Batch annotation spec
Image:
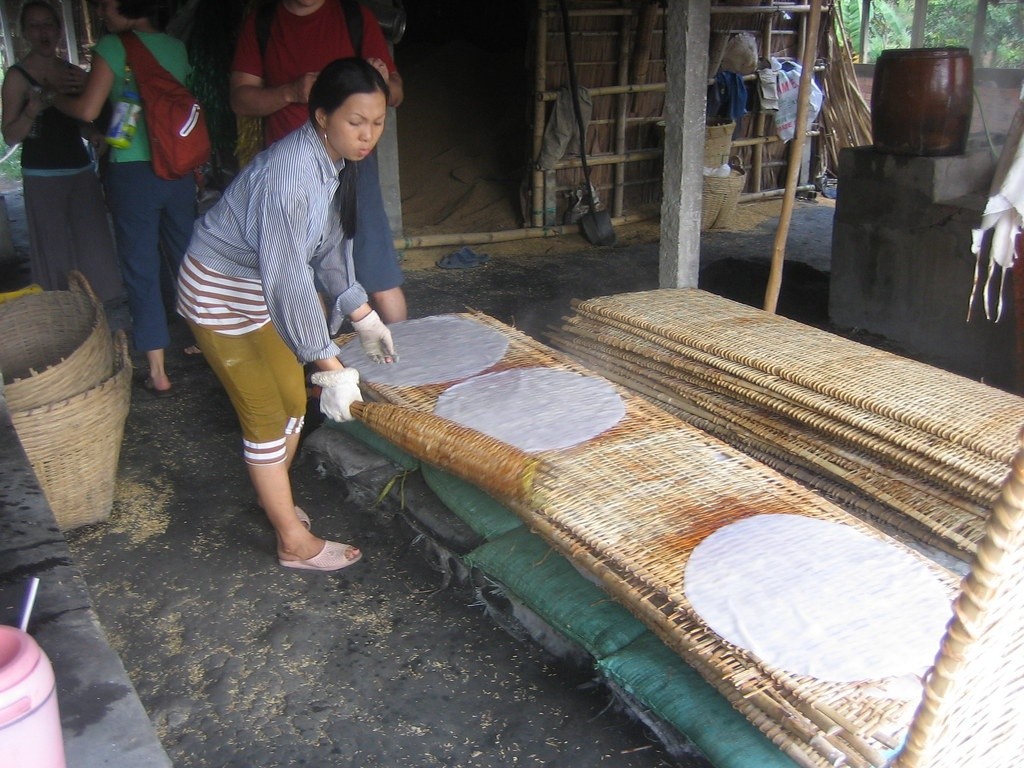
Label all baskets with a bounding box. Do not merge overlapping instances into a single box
[657,116,746,230]
[0,270,132,534]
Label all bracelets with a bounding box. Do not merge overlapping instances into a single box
[24,109,36,121]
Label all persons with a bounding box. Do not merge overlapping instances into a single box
[0,0,411,397]
[174,56,401,571]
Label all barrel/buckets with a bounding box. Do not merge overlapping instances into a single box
[870,46,976,157]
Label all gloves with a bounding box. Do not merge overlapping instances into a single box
[350,309,399,366]
[311,368,364,423]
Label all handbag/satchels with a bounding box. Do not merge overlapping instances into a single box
[118,30,211,181]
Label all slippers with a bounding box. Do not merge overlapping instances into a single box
[279,540,362,571]
[258,500,310,532]
[435,247,490,269]
[183,345,203,357]
[144,375,177,398]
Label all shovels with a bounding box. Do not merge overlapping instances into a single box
[559,2,615,246]
